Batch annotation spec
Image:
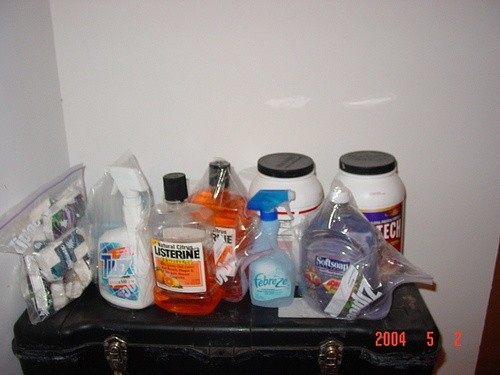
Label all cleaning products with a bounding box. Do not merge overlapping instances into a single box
[297,180,378,318]
[147,172,224,317]
[246,189,296,308]
[187,159,257,304]
[97,167,155,311]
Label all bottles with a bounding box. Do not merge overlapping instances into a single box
[333,150,406,279]
[247,152,324,286]
[149,172,221,316]
[298,189,378,319]
[189,160,249,302]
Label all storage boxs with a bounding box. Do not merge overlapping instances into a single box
[12,280,440,375]
